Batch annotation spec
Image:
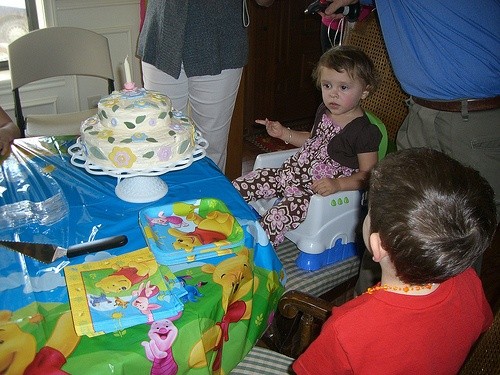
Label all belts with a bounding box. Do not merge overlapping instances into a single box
[411,95,500,112]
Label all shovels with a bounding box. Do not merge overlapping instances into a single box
[0,234,128,265]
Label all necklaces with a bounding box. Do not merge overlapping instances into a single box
[355,281,437,299]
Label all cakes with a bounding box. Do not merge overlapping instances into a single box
[79,87,195,169]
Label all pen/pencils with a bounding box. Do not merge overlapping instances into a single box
[255,120,267,126]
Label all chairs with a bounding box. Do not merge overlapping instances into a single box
[249,134,398,300]
[225,289,500,375]
[249,108,388,273]
[8,26,116,135]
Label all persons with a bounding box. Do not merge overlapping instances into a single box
[292,145,495,375]
[232,43,383,249]
[325,0,499,224]
[135,0,275,180]
[0,106,20,156]
[285,127,292,144]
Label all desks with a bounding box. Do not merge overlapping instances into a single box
[0,136,259,373]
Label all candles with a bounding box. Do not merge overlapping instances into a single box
[122,52,136,90]
